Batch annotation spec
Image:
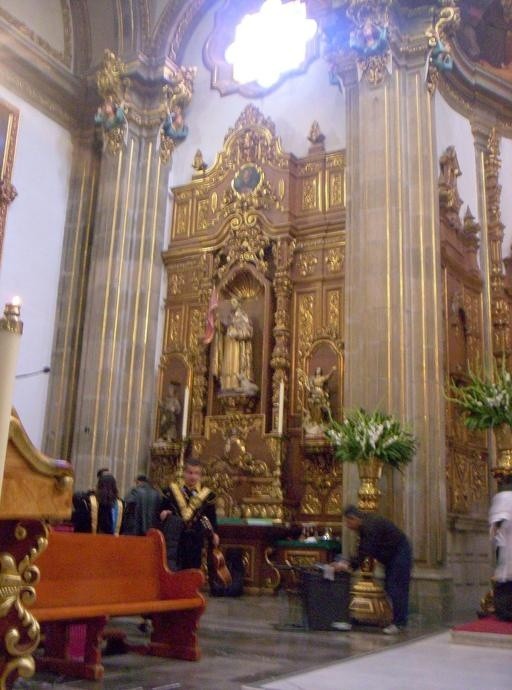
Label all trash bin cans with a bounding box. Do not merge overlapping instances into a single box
[300,566,351,631]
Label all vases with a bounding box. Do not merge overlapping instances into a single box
[357,457,385,512]
[491,423,512,478]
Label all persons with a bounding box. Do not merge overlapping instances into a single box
[217,295,256,389]
[71,468,111,531]
[326,506,412,633]
[160,456,221,572]
[488,476,512,620]
[79,474,126,536]
[125,471,161,536]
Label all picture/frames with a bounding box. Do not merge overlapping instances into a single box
[0,97,20,272]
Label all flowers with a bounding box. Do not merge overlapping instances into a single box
[441,350,512,432]
[319,394,421,475]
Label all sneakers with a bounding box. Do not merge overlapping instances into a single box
[383,624,400,634]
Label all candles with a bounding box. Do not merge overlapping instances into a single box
[182,385,189,440]
[278,379,284,434]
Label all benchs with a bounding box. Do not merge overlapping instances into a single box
[22,528,207,682]
[264,548,351,630]
